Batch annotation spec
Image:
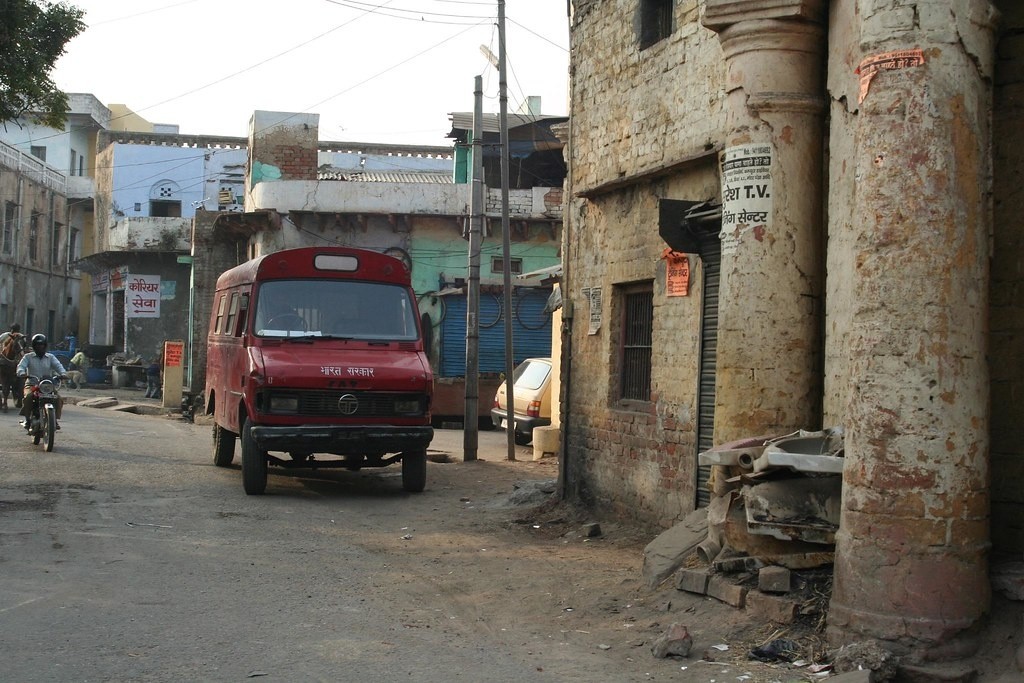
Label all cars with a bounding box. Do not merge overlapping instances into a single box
[490,356,553,448]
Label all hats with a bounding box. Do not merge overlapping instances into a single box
[8,323,21,330]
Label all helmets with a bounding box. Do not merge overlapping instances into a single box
[32,334,47,357]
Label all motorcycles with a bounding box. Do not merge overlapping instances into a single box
[19,373,68,452]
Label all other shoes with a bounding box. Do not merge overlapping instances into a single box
[23,420,29,428]
[56,421,60,429]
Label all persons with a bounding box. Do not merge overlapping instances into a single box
[15,334,68,430]
[69,349,87,389]
[144,364,161,398]
[261,292,304,331]
[0,323,26,354]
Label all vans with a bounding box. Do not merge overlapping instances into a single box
[204,245,435,497]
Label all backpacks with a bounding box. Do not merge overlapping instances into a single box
[7,333,27,360]
